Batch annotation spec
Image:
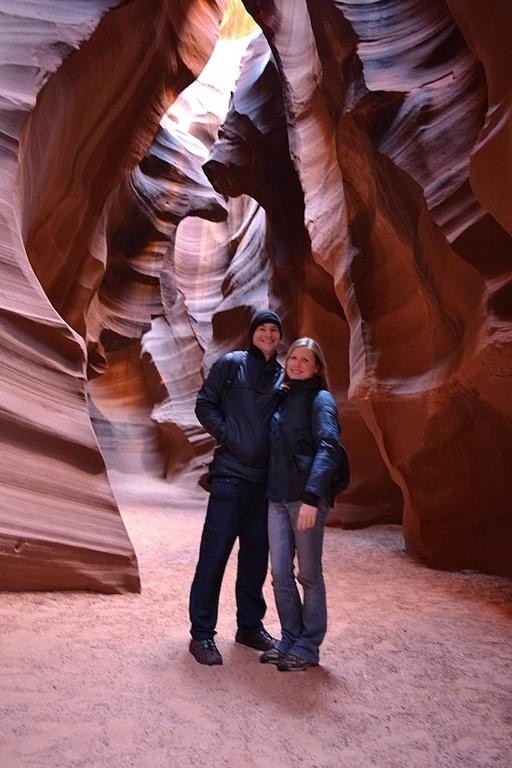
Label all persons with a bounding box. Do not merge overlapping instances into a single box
[260,337,349,671]
[190,308,280,664]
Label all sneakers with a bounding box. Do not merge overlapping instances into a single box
[190,638,222,665]
[260,649,319,671]
[236,631,277,649]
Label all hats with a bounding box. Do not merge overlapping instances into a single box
[250,310,283,340]
